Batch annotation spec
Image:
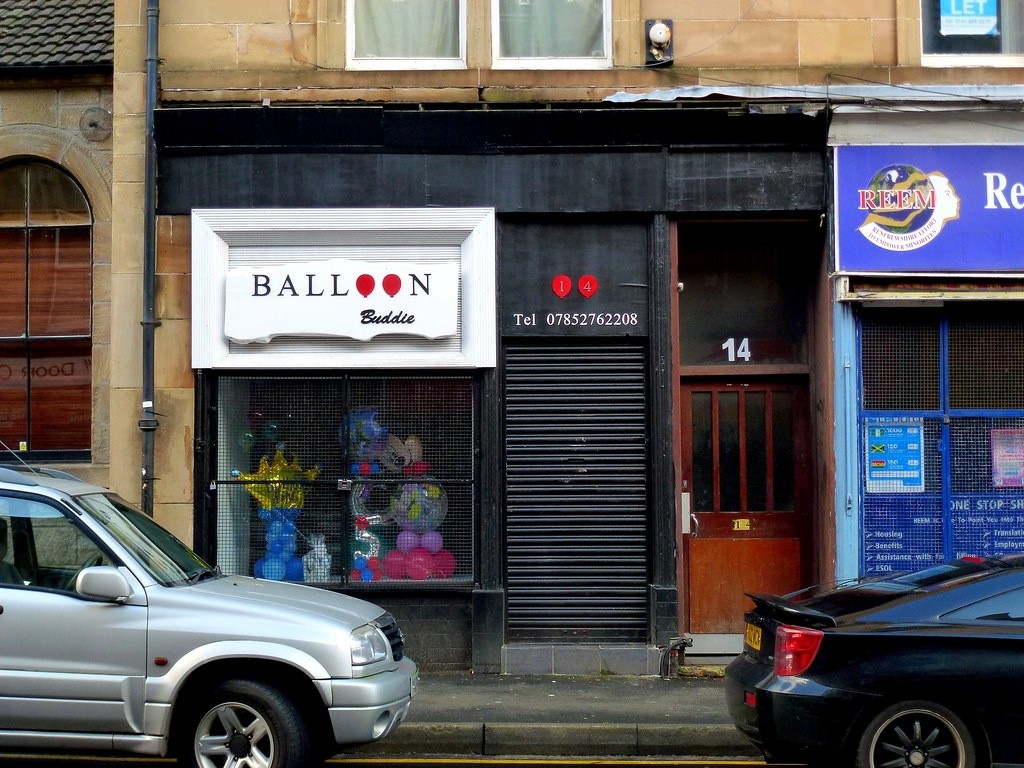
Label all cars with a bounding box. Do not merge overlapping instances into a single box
[724,553,1024,768]
[1,464,419,768]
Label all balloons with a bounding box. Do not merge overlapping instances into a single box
[232,445,321,581]
[338,406,455,580]
